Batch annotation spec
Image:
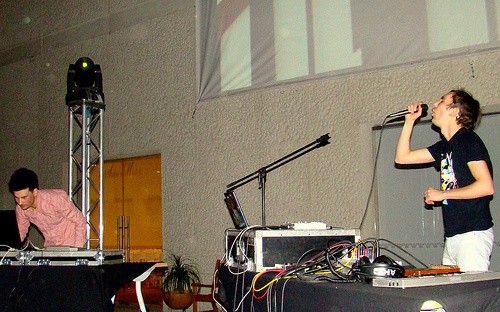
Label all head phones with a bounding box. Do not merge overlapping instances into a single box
[352,255,405,283]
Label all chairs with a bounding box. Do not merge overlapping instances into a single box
[190,259,221,312]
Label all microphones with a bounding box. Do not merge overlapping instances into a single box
[386,104,428,118]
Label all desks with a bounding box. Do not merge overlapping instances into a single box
[219,264,500,312]
[1,266,167,312]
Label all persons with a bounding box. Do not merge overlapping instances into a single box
[8,168,87,249]
[394,87,495,271]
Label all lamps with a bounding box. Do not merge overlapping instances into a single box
[67,56,104,95]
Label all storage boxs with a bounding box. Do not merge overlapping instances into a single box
[227,228,362,272]
[0,249,127,266]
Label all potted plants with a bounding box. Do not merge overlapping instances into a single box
[164,251,195,309]
